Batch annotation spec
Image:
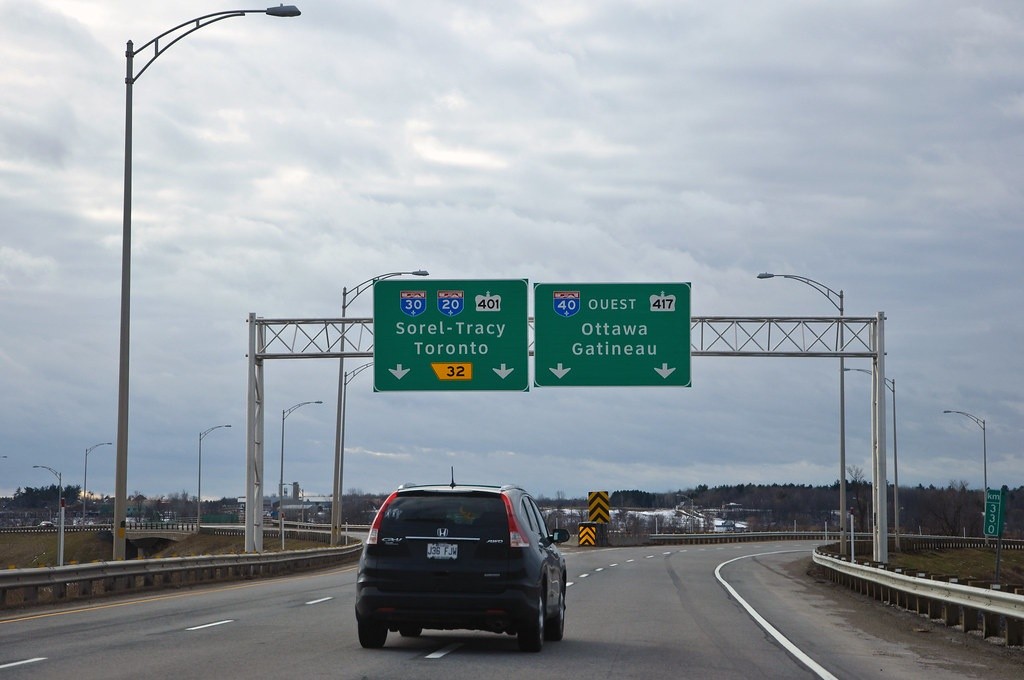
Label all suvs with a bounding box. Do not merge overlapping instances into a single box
[353,483,571,654]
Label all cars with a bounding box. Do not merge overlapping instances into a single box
[37,521,54,527]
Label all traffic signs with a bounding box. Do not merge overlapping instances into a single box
[370,278,533,393]
[529,280,693,388]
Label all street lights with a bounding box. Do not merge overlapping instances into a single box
[675,494,694,533]
[842,367,902,552]
[33,464,61,565]
[82,442,113,525]
[755,272,848,556]
[279,401,324,548]
[329,269,435,545]
[942,410,986,513]
[195,425,232,532]
[114,5,301,562]
